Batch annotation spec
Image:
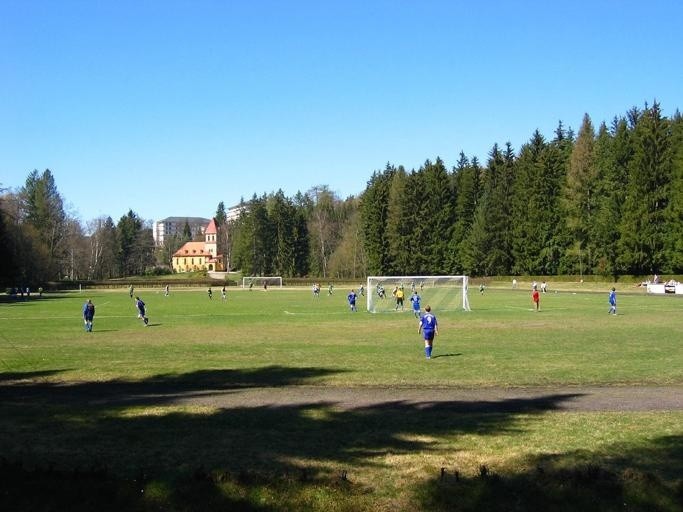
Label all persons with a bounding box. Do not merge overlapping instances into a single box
[417,305,438,359]
[532,279,537,292]
[312,283,320,299]
[134,297,148,325]
[540,281,546,293]
[409,291,421,318]
[37,287,43,296]
[511,278,516,289]
[347,289,356,311]
[129,284,133,297]
[375,279,425,301]
[220,285,227,300]
[327,282,334,296]
[607,287,618,314]
[207,287,212,300]
[394,288,404,311]
[82,299,93,332]
[358,282,364,297]
[164,284,168,296]
[532,288,540,312]
[479,282,484,294]
[249,282,254,291]
[262,282,266,292]
